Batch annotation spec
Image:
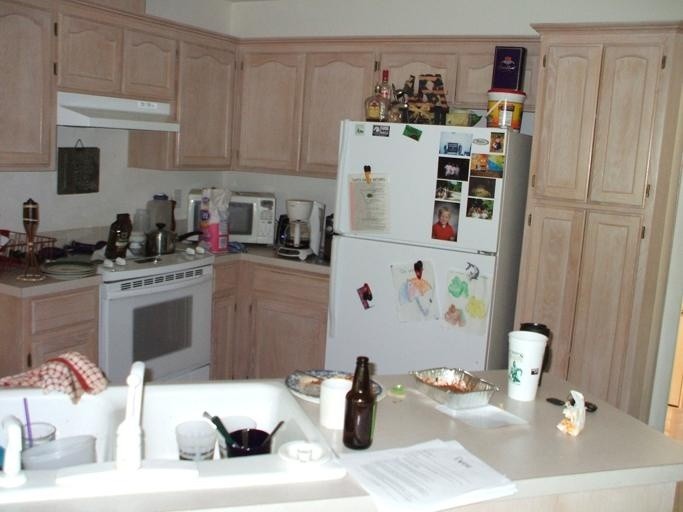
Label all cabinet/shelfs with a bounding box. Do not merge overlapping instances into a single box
[125,22,239,172]
[236,35,379,181]
[510,20,681,427]
[55,0,181,123]
[374,34,541,114]
[211,259,329,382]
[0,1,57,173]
[0,285,102,380]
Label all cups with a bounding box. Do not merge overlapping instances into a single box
[175,419,218,464]
[506,329,549,403]
[22,420,58,452]
[317,375,348,434]
[217,421,273,458]
[517,317,549,393]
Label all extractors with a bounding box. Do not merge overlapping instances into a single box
[54,90,180,132]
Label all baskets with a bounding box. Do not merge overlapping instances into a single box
[1,229,58,272]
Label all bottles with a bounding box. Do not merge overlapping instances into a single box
[343,357,378,452]
[144,191,174,230]
[134,208,150,230]
[363,69,392,121]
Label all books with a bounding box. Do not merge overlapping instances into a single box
[345,437,517,511]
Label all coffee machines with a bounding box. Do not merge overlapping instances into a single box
[276,198,324,258]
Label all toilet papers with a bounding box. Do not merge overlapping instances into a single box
[319,378,353,432]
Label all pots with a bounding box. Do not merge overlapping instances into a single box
[144,224,203,255]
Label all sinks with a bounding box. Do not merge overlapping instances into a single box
[1,379,348,508]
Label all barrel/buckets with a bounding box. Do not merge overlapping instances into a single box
[146,193,174,232]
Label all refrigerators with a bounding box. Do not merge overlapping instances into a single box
[322,119,532,380]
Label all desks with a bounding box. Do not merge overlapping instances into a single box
[279,367,681,512]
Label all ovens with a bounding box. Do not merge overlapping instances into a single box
[100,267,213,380]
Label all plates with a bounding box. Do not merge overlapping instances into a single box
[40,261,96,281]
[284,368,387,407]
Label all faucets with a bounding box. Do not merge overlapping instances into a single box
[116,360,146,472]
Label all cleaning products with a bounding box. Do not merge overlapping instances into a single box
[197,186,232,252]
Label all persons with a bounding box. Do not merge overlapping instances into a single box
[431,136,503,243]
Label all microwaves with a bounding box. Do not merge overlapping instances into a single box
[186,184,276,249]
